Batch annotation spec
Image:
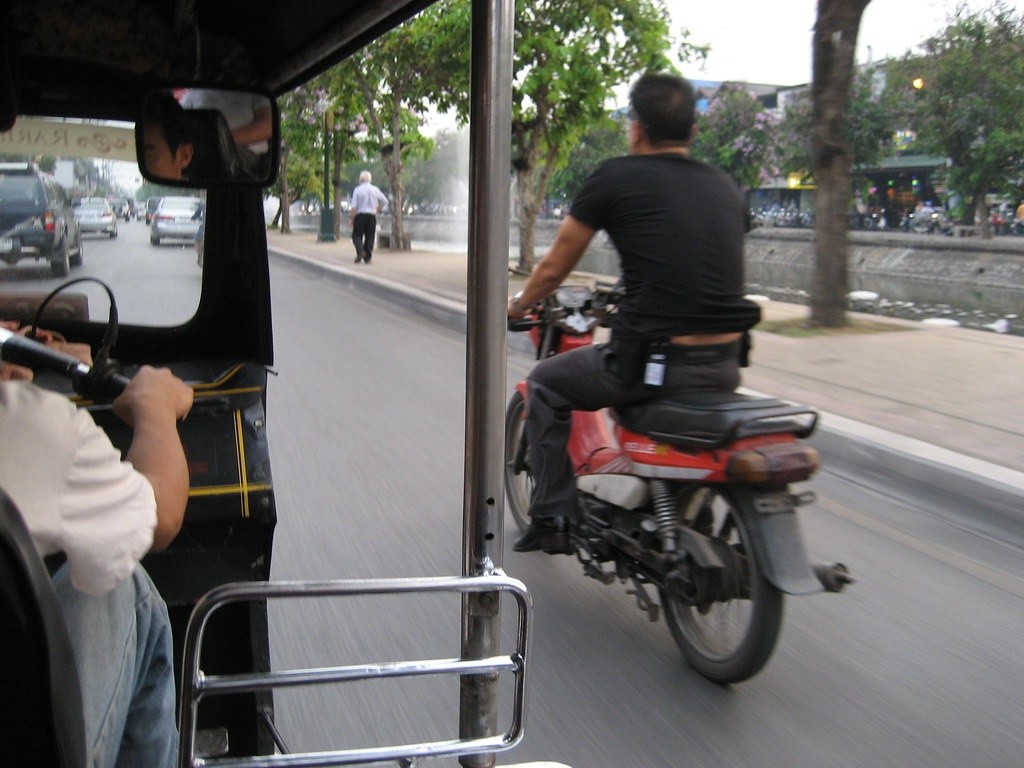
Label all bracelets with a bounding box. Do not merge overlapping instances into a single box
[510,289,535,312]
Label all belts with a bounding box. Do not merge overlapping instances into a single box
[611,339,742,364]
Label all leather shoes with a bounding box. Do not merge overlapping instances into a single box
[512,515,570,551]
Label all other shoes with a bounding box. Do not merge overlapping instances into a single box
[355,250,370,262]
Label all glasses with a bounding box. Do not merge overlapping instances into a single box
[142,146,177,167]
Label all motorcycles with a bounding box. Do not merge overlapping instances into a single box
[506,280,856,685]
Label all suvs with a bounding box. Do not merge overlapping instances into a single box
[0,160,84,278]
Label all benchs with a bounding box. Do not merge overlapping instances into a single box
[953,225,996,239]
[376,230,411,251]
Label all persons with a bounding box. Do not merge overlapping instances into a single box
[348,170,388,265]
[507,73,761,553]
[191,189,206,265]
[0,364,193,767]
[756,186,1024,233]
[141,89,272,181]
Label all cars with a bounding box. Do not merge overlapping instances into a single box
[109,196,146,223]
[145,198,161,224]
[149,195,202,247]
[70,197,118,239]
